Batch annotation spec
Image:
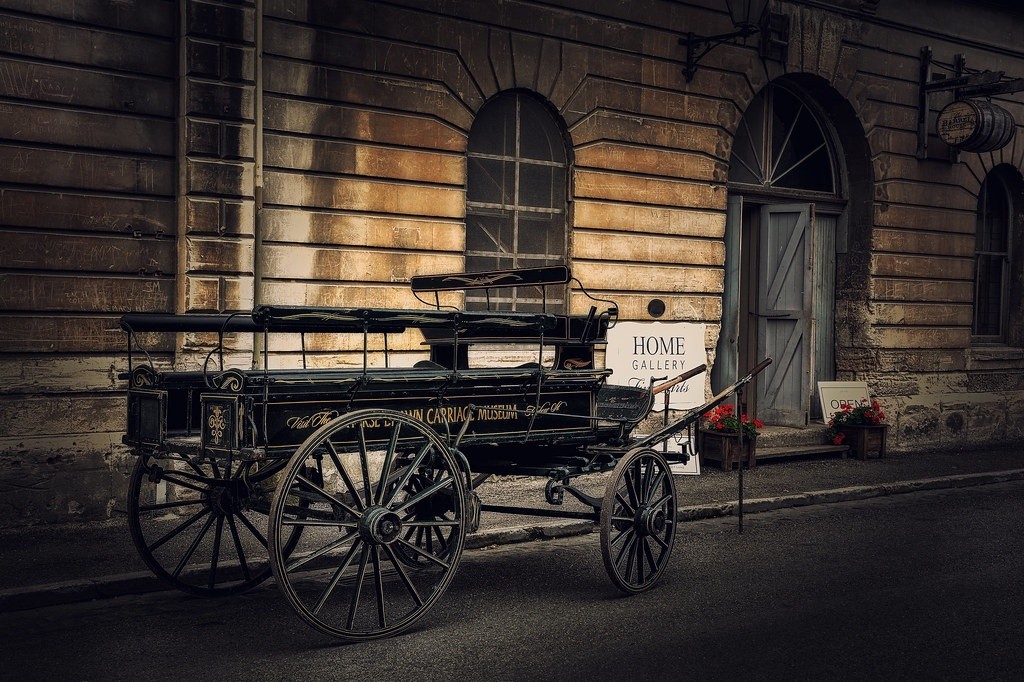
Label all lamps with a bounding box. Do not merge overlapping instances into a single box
[678,0,770,84]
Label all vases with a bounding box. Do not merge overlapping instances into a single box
[696,429,761,472]
[837,424,890,461]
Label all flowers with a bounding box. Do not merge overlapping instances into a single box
[703,405,763,434]
[825,399,885,446]
